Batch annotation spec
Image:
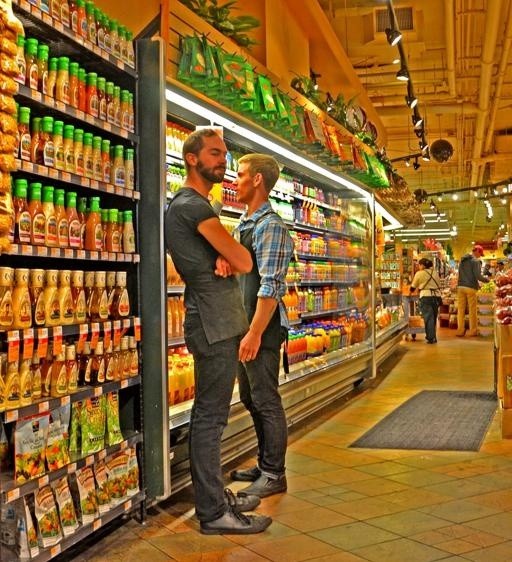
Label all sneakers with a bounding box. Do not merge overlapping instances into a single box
[230,464,261,481]
[426,336,436,344]
[223,488,261,511]
[237,474,287,498]
[200,508,272,535]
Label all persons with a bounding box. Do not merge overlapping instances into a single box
[497,262,504,277]
[225,154,295,499]
[165,129,272,535]
[484,264,492,276]
[410,258,442,344]
[456,246,490,338]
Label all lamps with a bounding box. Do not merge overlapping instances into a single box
[418,140,427,150]
[429,193,443,222]
[412,156,421,170]
[405,95,419,109]
[396,64,409,81]
[385,27,403,46]
[411,114,423,127]
[422,153,430,162]
[414,129,425,138]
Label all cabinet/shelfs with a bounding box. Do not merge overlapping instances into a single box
[166,112,371,498]
[375,195,455,374]
[0,0,150,562]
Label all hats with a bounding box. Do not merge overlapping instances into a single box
[473,245,484,256]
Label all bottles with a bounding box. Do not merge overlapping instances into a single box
[10,92,134,192]
[0,266,129,319]
[8,0,136,69]
[375,302,405,334]
[1,170,136,255]
[11,7,133,131]
[0,335,140,411]
[167,120,368,407]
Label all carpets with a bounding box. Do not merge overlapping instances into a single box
[347,390,497,452]
